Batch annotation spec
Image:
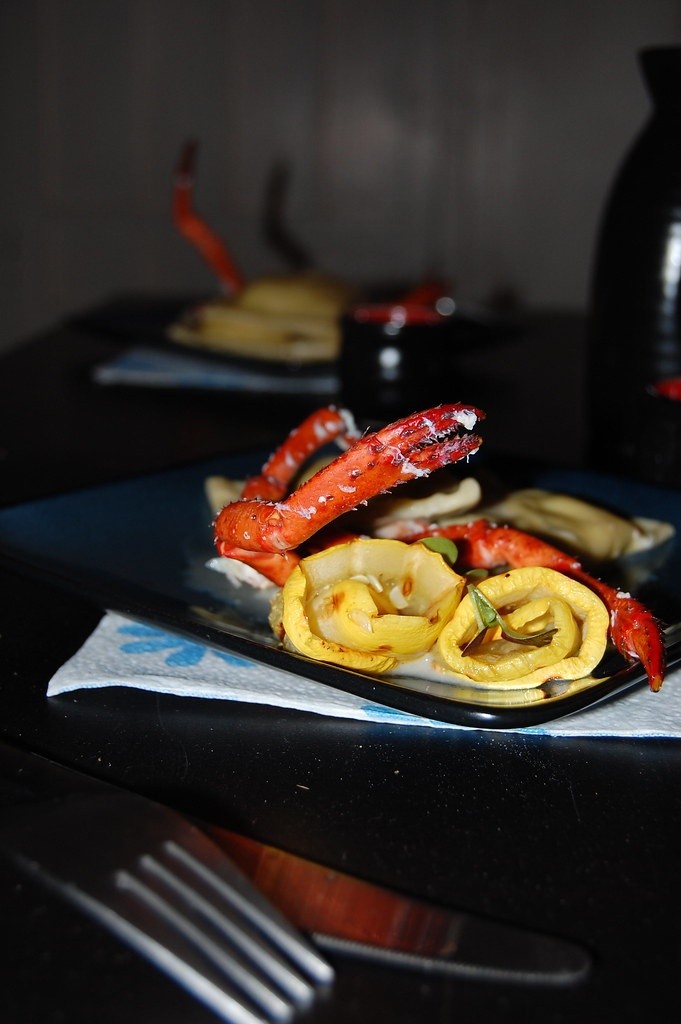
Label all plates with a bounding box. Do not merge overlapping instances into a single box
[66,294,336,374]
[0,451,681,726]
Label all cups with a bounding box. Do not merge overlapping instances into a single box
[333,281,516,498]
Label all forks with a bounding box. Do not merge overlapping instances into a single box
[0,746,334,1023]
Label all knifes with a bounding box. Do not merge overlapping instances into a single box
[192,820,591,978]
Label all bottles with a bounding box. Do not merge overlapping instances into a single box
[586,38,681,475]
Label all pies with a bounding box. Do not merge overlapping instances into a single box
[194,277,350,346]
[282,539,466,674]
[441,560,608,687]
